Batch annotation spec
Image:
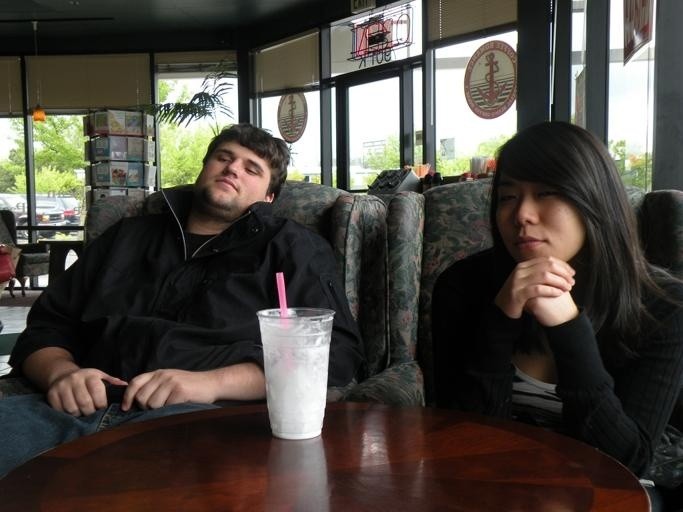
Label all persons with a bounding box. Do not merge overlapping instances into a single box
[427,121,681,511]
[0,123,367,480]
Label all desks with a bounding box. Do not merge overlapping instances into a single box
[83,410,647,495]
[0,398,652,512]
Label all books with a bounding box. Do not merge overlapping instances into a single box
[80,108,159,210]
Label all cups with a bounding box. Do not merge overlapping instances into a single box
[255,306,337,441]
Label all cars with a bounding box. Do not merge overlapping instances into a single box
[0,192,82,241]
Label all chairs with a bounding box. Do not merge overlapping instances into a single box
[385,180,683,400]
[0,217,50,295]
[83,194,381,400]
